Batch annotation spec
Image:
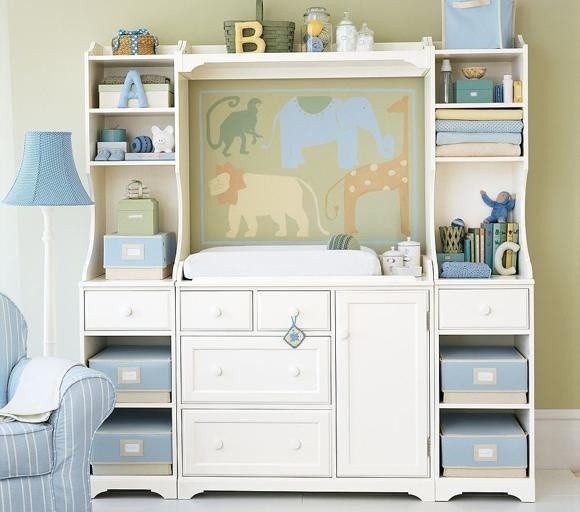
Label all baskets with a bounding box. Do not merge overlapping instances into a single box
[223,0,296,54]
[112,32,159,55]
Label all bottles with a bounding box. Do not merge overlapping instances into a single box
[439,58,454,103]
[381,235,423,279]
[302,7,332,52]
[335,12,376,52]
[502,73,513,103]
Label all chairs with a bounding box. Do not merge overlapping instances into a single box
[0,291,116,512]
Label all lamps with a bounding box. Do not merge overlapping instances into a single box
[1,130,95,358]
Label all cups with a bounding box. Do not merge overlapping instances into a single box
[101,129,126,141]
[438,225,464,253]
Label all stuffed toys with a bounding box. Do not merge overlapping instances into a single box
[480,190,516,223]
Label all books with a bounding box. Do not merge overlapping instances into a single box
[466,223,519,275]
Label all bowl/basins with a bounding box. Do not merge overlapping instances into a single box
[461,67,488,79]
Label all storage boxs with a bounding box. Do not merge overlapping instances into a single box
[441,0,517,50]
